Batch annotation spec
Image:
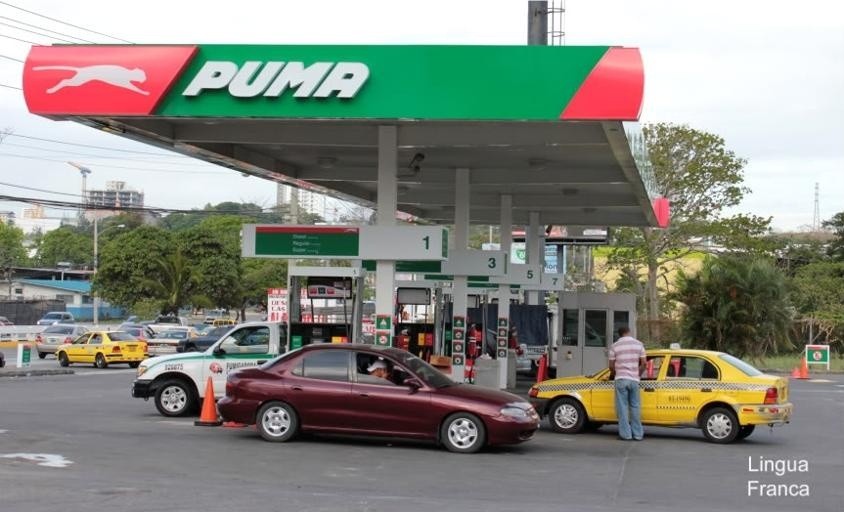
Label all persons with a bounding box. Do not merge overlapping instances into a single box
[607,327,649,442]
[383,359,405,384]
[367,361,389,381]
[467,315,488,347]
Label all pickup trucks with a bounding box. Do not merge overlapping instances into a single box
[131,319,297,417]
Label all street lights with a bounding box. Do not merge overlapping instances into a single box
[92,224,126,326]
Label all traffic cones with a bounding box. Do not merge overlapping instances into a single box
[192,376,223,427]
[796,357,811,379]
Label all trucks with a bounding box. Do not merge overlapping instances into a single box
[467,305,603,376]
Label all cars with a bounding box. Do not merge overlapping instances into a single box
[483,328,533,373]
[527,342,794,446]
[0,315,14,327]
[216,342,542,453]
[34,310,239,370]
